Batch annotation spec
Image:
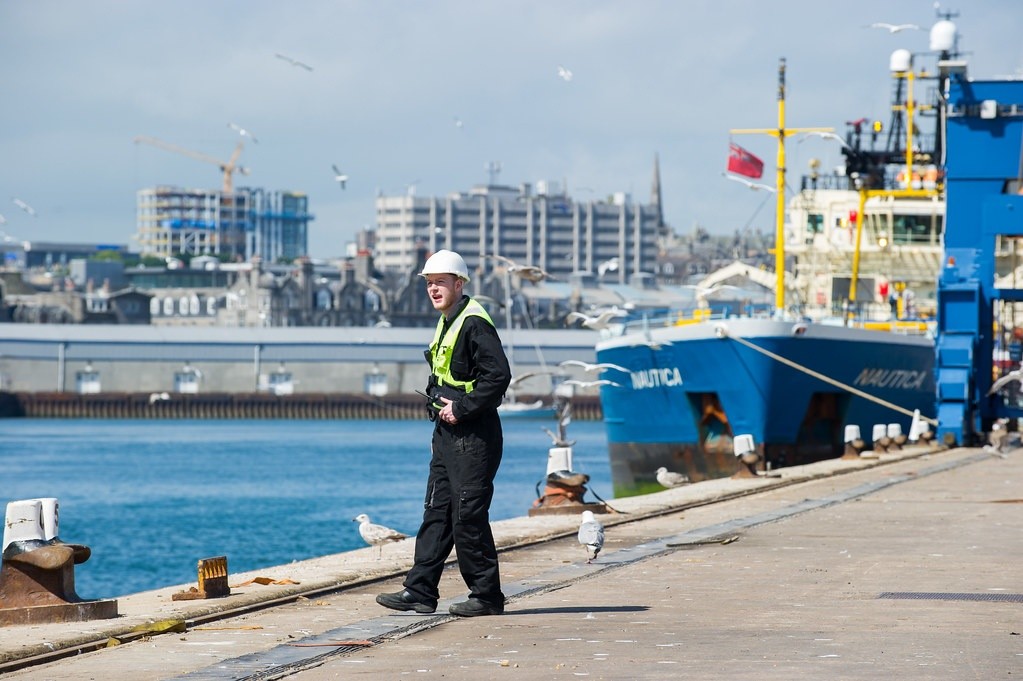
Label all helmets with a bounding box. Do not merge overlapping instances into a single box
[416,249,470,286]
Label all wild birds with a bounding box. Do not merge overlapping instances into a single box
[332,164,349,190]
[864,22,931,36]
[556,358,634,375]
[557,66,572,81]
[226,121,258,143]
[148,392,170,405]
[352,513,412,561]
[559,378,624,391]
[562,310,620,330]
[654,467,691,489]
[478,253,559,286]
[13,199,37,218]
[722,173,777,195]
[797,132,855,153]
[507,371,536,391]
[679,283,740,300]
[276,53,313,72]
[597,257,621,276]
[632,339,675,352]
[577,510,606,564]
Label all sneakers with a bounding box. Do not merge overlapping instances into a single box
[375,589,437,613]
[449,597,504,615]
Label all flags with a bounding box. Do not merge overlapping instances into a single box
[727,142,764,179]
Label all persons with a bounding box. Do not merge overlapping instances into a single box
[376,249,511,618]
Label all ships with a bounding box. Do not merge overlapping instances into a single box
[595,0,1022,500]
